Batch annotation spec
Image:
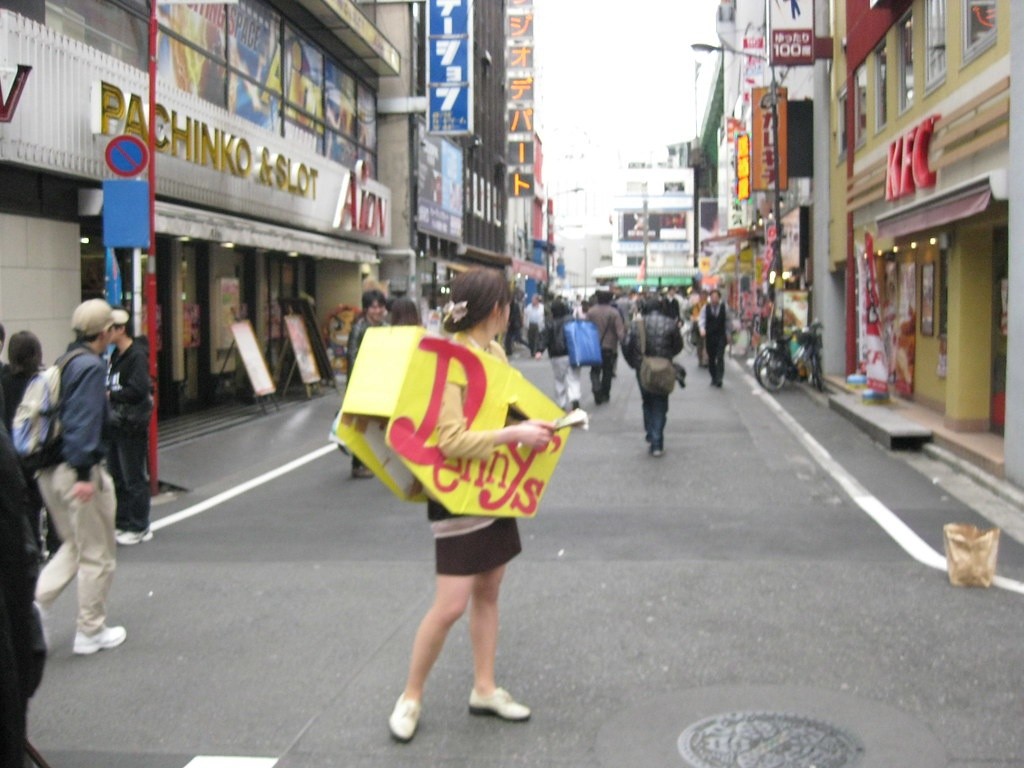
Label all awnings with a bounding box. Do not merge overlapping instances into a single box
[77,187,382,266]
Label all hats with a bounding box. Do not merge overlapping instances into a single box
[71,299,129,336]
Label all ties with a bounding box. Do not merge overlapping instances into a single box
[712,306,717,315]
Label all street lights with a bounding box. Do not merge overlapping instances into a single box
[690,43,784,338]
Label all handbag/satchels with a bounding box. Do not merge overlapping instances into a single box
[640,357,676,395]
[565,308,603,368]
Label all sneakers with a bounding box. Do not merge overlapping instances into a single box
[74,626,127,655]
[469,687,532,721]
[117,526,154,545]
[390,694,420,741]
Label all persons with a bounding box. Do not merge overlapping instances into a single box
[690,291,729,387]
[390,270,556,742]
[502,289,545,361]
[549,286,619,411]
[619,285,687,456]
[111,308,152,545]
[0,299,125,768]
[346,289,422,478]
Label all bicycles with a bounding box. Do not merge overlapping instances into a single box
[752,317,824,392]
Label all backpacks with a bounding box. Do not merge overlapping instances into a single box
[12,349,88,458]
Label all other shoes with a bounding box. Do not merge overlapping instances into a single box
[595,395,610,405]
[352,467,374,479]
[712,380,722,387]
[652,444,662,456]
[646,434,652,441]
[572,400,579,409]
